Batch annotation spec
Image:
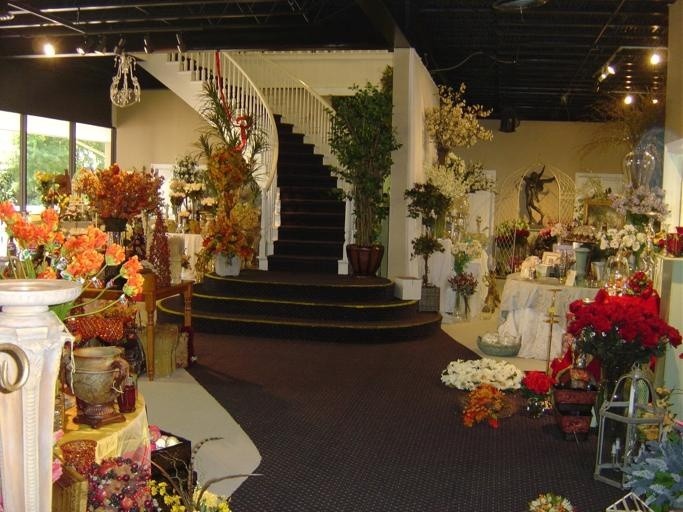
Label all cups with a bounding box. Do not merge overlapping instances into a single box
[529,269,538,281]
[559,264,566,282]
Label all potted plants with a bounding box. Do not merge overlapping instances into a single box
[323,64,404,280]
[404,182,454,312]
[193,80,273,182]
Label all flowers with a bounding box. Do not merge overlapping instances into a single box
[494,218,530,279]
[450,240,483,275]
[560,415,591,442]
[623,435,683,511]
[564,287,683,381]
[440,357,525,393]
[534,223,563,259]
[557,387,597,415]
[88,457,154,512]
[150,437,266,512]
[0,201,145,323]
[522,370,558,397]
[599,226,647,262]
[423,82,499,239]
[167,145,261,266]
[460,383,517,427]
[529,495,574,512]
[638,385,683,442]
[448,272,477,299]
[613,185,672,223]
[32,165,166,225]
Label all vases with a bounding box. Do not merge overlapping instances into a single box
[463,422,500,468]
[525,396,554,420]
[101,218,128,250]
[454,295,468,323]
[215,250,241,278]
[598,365,625,449]
